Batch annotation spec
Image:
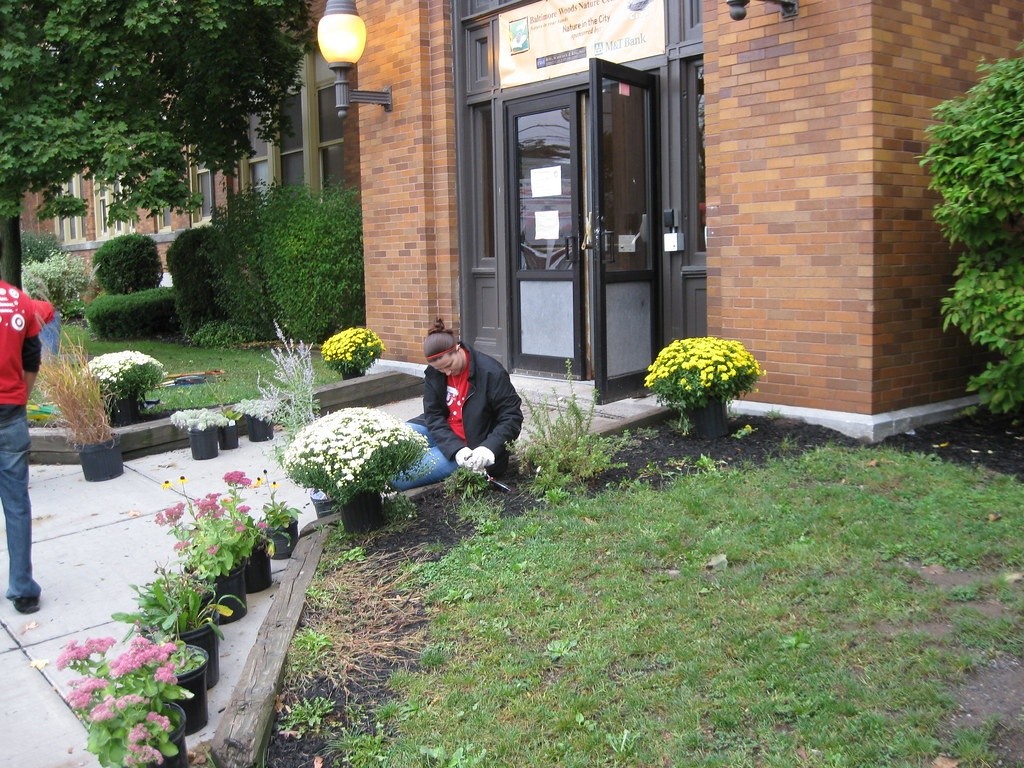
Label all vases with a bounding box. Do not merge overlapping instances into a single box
[248,419,274,442]
[108,388,139,424]
[335,491,385,534]
[342,373,366,380]
[180,556,248,625]
[163,644,210,736]
[186,428,220,461]
[261,519,299,560]
[245,541,272,595]
[74,434,123,482]
[139,609,220,691]
[120,701,189,768]
[310,488,340,520]
[693,395,729,439]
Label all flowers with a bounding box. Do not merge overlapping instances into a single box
[76,350,168,413]
[231,398,280,422]
[170,407,230,432]
[255,319,440,515]
[54,469,301,768]
[644,336,767,411]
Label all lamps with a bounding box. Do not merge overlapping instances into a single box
[318,0,392,120]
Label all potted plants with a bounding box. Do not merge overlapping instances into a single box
[216,407,243,449]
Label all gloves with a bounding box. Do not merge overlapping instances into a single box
[455,446,495,474]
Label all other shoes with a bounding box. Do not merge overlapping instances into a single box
[13,594,40,614]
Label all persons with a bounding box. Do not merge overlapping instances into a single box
[369,317,523,490]
[0,240,43,614]
[30,299,60,364]
